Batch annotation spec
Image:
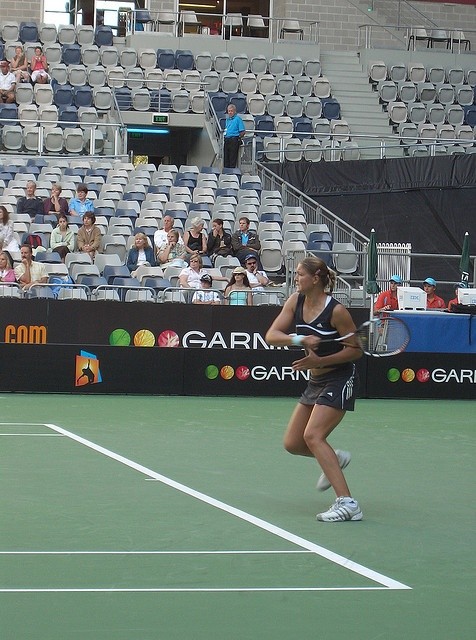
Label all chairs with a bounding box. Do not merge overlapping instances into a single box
[446,28,471,53]
[131,8,155,31]
[426,28,452,50]
[155,9,175,33]
[247,14,269,37]
[222,13,244,38]
[1,41,332,94]
[367,59,476,156]
[4,23,114,44]
[406,26,432,52]
[209,95,361,160]
[176,10,202,35]
[0,160,361,303]
[280,18,303,40]
[0,87,209,157]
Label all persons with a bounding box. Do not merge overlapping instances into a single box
[12,46,30,83]
[178,254,230,303]
[245,254,269,288]
[0,61,16,103]
[231,217,263,271]
[224,266,252,306]
[0,206,13,249]
[192,274,220,305]
[14,244,49,290]
[0,252,15,282]
[373,275,401,324]
[51,214,74,261]
[77,211,101,260]
[44,185,68,215]
[68,186,94,217]
[31,46,51,84]
[224,104,245,168]
[127,233,158,277]
[448,282,465,312]
[154,216,184,252]
[422,278,446,308]
[184,217,207,263]
[156,229,187,272]
[207,218,231,263]
[17,181,42,223]
[266,257,364,522]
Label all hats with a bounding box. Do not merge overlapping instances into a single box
[199,274,211,286]
[0,62,9,67]
[422,278,436,287]
[232,267,246,275]
[244,255,257,260]
[453,282,464,288]
[389,275,401,284]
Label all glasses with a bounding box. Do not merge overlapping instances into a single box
[189,260,196,262]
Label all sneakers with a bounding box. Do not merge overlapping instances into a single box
[315,496,363,521]
[316,449,349,492]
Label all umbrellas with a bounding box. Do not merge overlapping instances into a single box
[459,232,472,288]
[366,228,380,351]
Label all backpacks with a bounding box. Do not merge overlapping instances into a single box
[26,235,42,249]
